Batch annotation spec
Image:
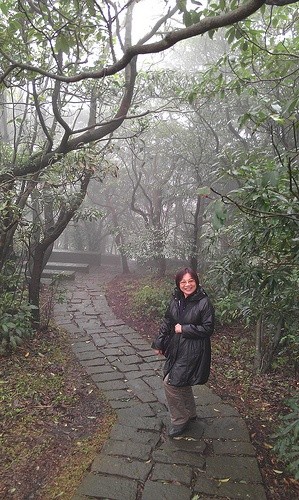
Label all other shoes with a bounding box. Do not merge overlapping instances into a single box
[169,422,186,437]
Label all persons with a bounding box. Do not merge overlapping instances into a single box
[151,267,215,438]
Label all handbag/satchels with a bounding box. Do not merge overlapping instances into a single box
[161,327,176,358]
